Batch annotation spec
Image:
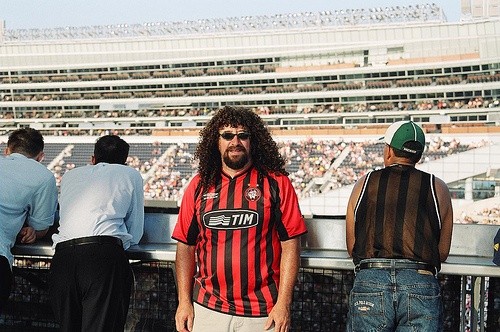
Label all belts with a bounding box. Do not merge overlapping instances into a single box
[55,236,121,250]
[360,262,436,271]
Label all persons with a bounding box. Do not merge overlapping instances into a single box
[346,120,453,332]
[0,93,494,221]
[48,135,144,332]
[172,106,308,332]
[0,128,58,315]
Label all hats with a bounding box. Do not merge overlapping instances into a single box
[375,120,425,154]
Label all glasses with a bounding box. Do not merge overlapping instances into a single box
[220,132,250,140]
